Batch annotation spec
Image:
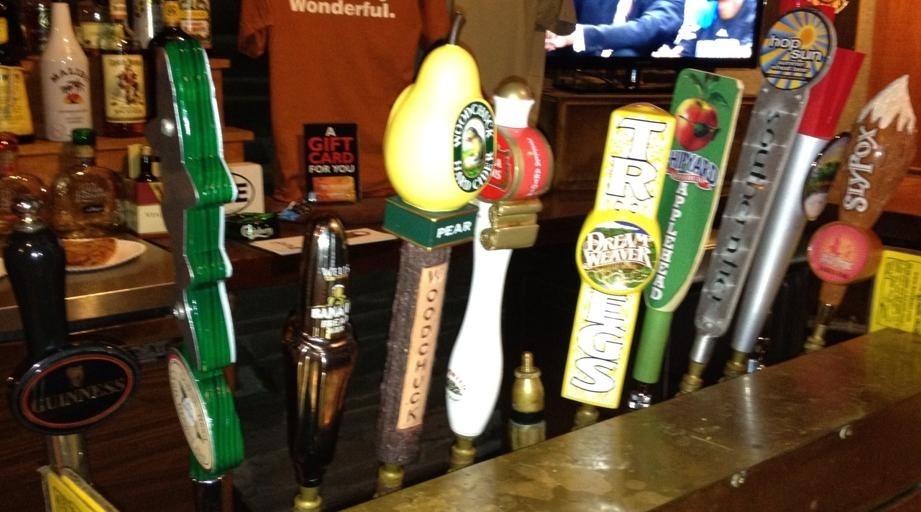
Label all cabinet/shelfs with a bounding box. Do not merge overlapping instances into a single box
[0,52,256,233]
[534,83,758,223]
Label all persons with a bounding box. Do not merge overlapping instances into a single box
[545,0,685,57]
[682,0,756,58]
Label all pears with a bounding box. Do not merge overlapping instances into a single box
[382,13,498,213]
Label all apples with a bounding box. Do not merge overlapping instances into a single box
[674,97,718,151]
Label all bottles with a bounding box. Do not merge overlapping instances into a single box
[98,0,148,136]
[41,3,95,144]
[160,1,215,58]
[135,145,160,183]
[0,0,36,145]
[54,126,127,239]
[0,129,56,256]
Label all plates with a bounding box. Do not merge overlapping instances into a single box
[62,237,148,274]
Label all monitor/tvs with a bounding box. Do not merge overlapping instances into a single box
[545,0,763,94]
